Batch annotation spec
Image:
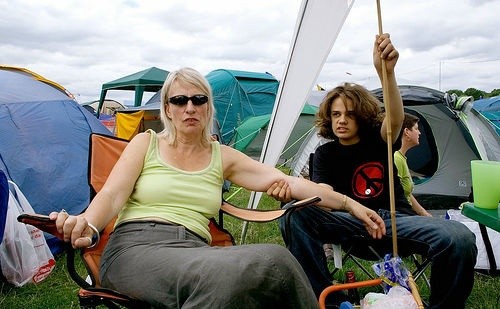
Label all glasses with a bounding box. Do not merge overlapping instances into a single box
[169,94,208,106]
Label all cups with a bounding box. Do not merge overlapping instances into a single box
[471,159,500,210]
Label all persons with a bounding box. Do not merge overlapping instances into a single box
[267,34,478,309]
[49,67,386,309]
[393,113,435,218]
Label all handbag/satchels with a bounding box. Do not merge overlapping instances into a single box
[0,180,56,287]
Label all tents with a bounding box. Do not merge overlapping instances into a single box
[286,85,500,218]
[203,69,280,146]
[114,87,232,193]
[0,68,114,275]
[77,99,127,122]
[96,67,171,119]
[226,103,320,167]
[470,94,500,136]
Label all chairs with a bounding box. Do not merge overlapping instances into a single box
[16,132,431,309]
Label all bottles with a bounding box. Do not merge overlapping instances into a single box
[345,271,360,305]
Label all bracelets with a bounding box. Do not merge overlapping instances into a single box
[342,193,349,212]
[83,222,101,249]
